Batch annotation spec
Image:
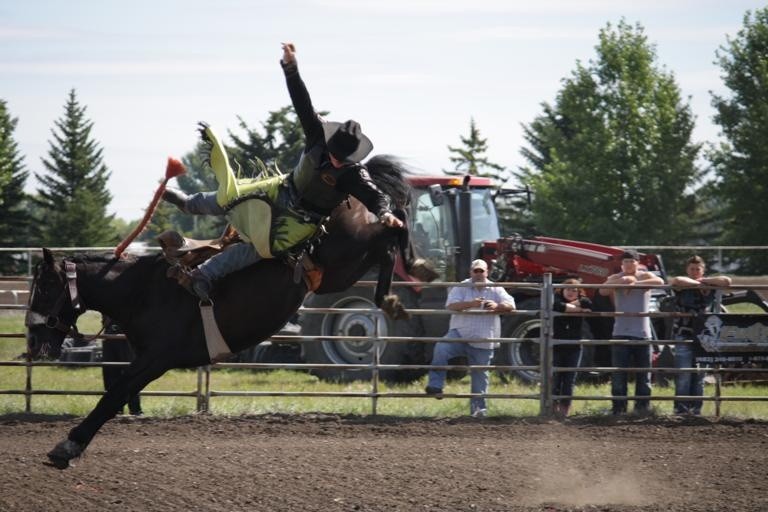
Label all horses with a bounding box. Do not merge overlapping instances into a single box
[23,152,443,471]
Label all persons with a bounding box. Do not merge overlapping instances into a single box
[162,42,404,303]
[553,279,593,417]
[598,250,664,415]
[426,259,516,418]
[671,255,731,414]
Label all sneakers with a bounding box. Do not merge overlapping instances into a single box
[161,187,187,214]
[552,403,571,417]
[191,267,209,302]
[424,385,443,399]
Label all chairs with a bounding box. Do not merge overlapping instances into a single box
[415,223,448,267]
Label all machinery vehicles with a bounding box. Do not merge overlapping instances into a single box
[228,165,768,395]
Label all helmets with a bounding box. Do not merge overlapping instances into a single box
[470,258,489,273]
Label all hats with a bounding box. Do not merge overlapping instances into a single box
[621,250,640,261]
[320,120,374,165]
